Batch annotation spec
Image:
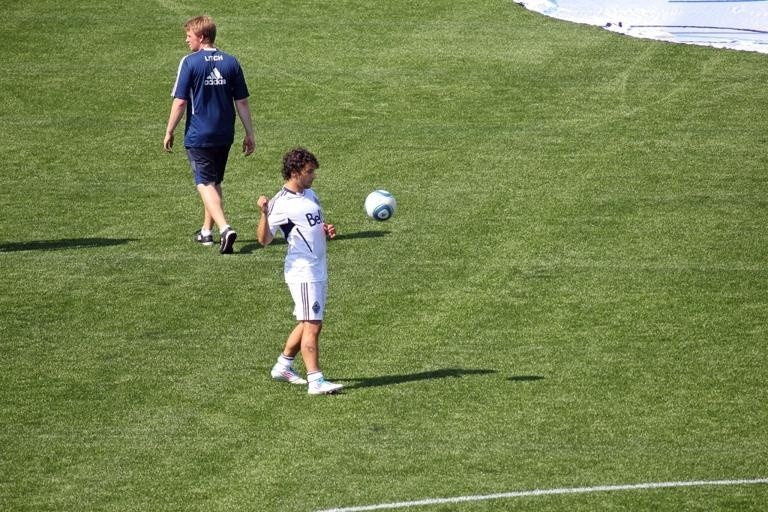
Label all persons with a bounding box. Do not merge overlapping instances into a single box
[258,147,345,395]
[162,15,256,255]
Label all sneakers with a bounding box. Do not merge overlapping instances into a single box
[308,382,343,394]
[219,227,237,254]
[271,365,308,385]
[194,229,214,245]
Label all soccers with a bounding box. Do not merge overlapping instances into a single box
[365,190,397,221]
